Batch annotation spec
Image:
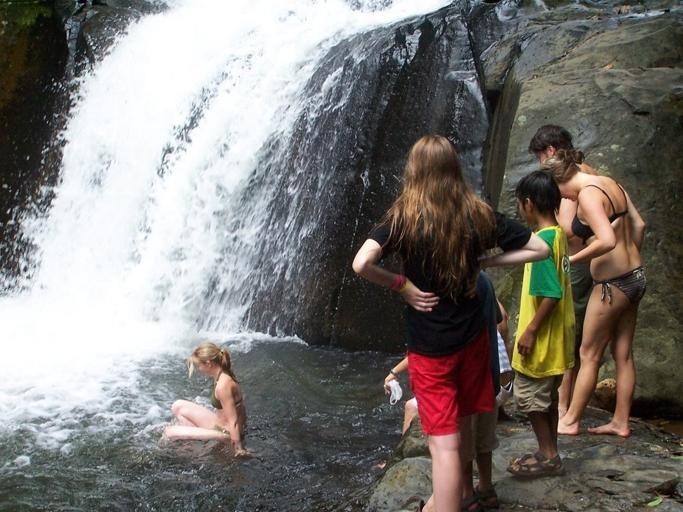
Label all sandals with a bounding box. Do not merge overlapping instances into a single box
[506,451,565,477]
[461,484,499,512]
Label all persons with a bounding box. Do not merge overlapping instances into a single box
[460,271,505,512]
[542,146,646,438]
[159,342,251,457]
[382,330,514,436]
[349,132,548,512]
[507,166,576,479]
[527,126,593,420]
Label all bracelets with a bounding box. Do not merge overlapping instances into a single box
[389,274,411,294]
[389,369,397,376]
